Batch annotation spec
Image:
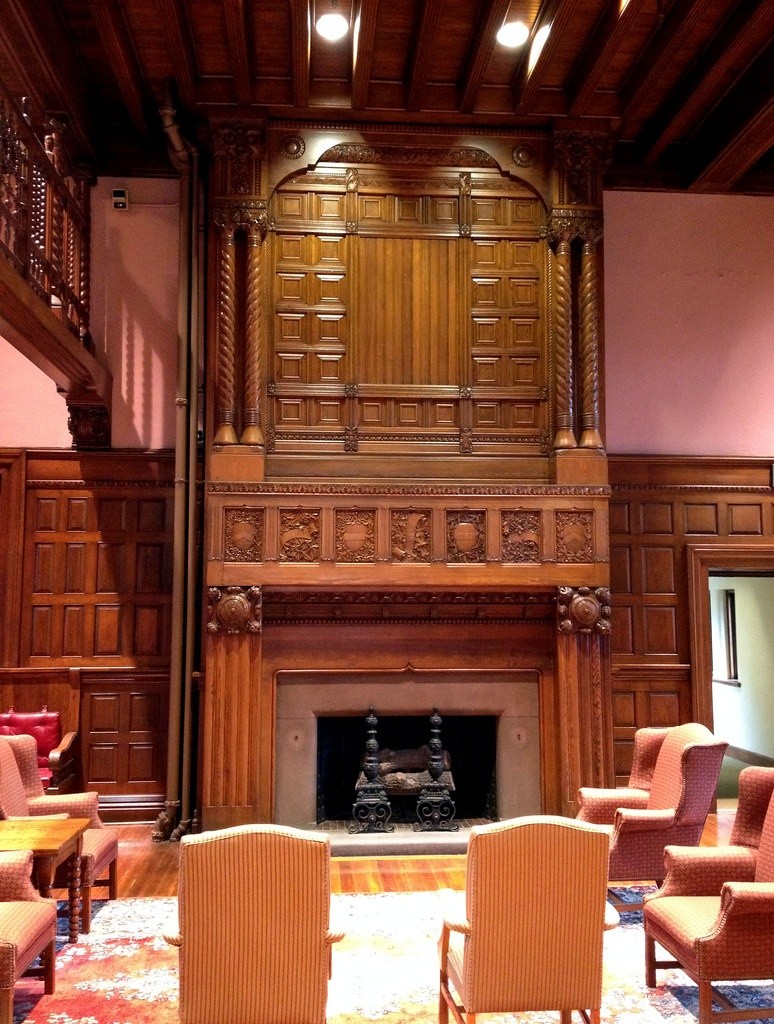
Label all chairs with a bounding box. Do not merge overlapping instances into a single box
[0,734,120,936]
[642,766,774,1024]
[0,668,83,796]
[0,850,56,1024]
[573,723,730,882]
[163,824,346,1024]
[438,815,613,1024]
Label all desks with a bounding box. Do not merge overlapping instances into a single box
[0,816,94,969]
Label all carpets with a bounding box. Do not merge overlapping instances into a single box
[11,884,774,1024]
